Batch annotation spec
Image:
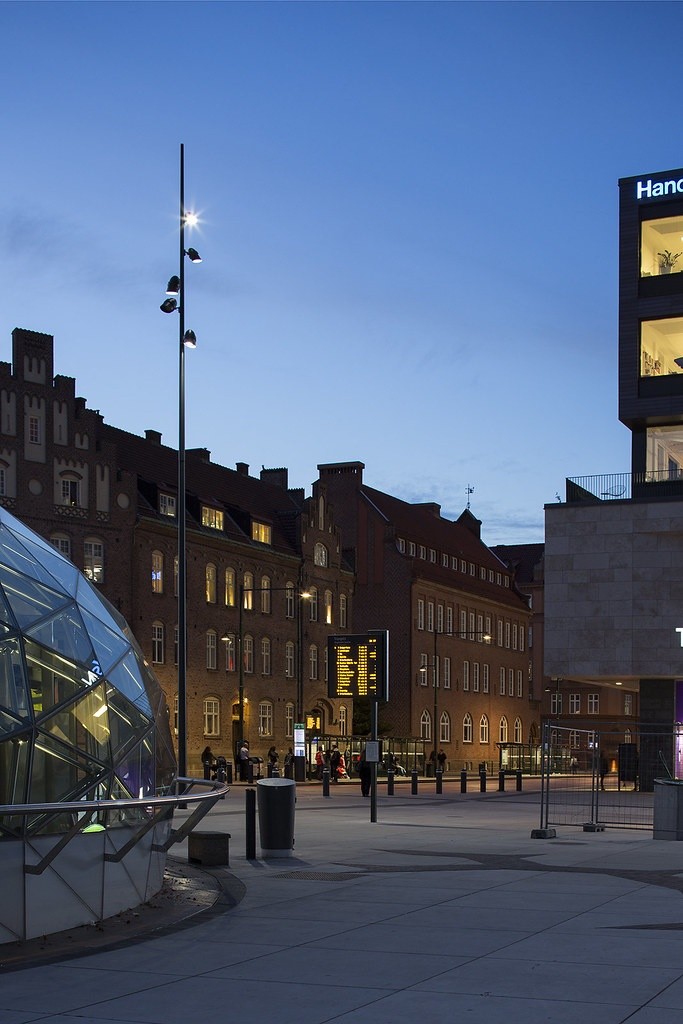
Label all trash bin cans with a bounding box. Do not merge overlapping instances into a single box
[289,756,306,782]
[257,778,298,858]
[651,778,683,841]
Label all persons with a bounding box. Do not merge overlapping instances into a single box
[240,742,249,782]
[569,755,577,774]
[429,749,447,774]
[201,746,219,780]
[311,745,351,784]
[382,749,408,778]
[267,746,294,778]
[599,749,609,791]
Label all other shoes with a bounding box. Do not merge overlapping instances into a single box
[331,780,338,784]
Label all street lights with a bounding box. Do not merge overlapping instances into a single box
[236,584,310,770]
[159,144,202,809]
[545,678,559,749]
[433,628,491,777]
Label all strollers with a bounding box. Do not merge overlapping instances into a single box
[338,765,350,780]
[395,762,408,777]
[211,756,228,780]
